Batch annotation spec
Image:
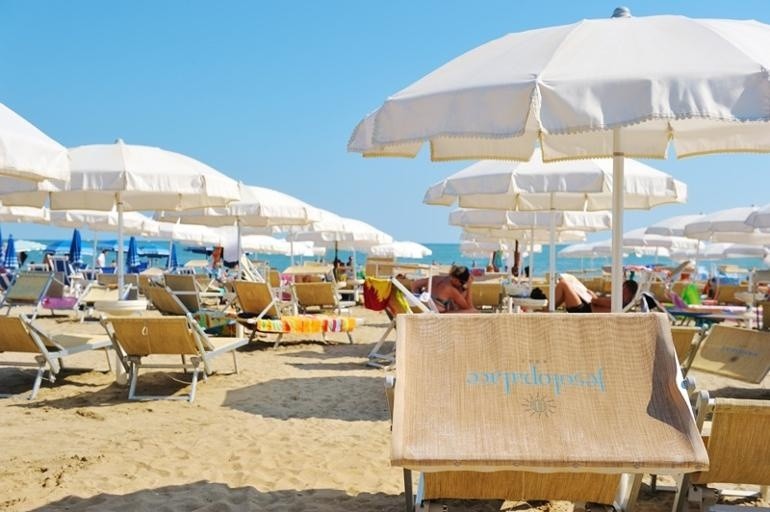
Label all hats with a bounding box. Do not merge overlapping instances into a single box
[453,267,469,281]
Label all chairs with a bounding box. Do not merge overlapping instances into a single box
[0,250,355,405]
[357,259,768,511]
